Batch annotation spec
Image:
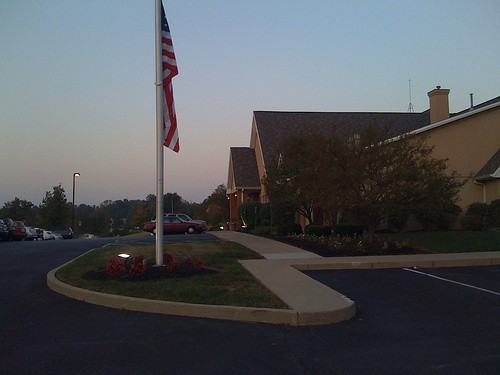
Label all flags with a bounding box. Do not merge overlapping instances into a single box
[161,0,180,153]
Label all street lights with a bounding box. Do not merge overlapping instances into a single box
[71,172,81,231]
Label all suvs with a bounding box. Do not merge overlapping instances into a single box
[151,213,207,232]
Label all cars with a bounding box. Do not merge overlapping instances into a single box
[144,217,203,234]
[1,218,74,240]
